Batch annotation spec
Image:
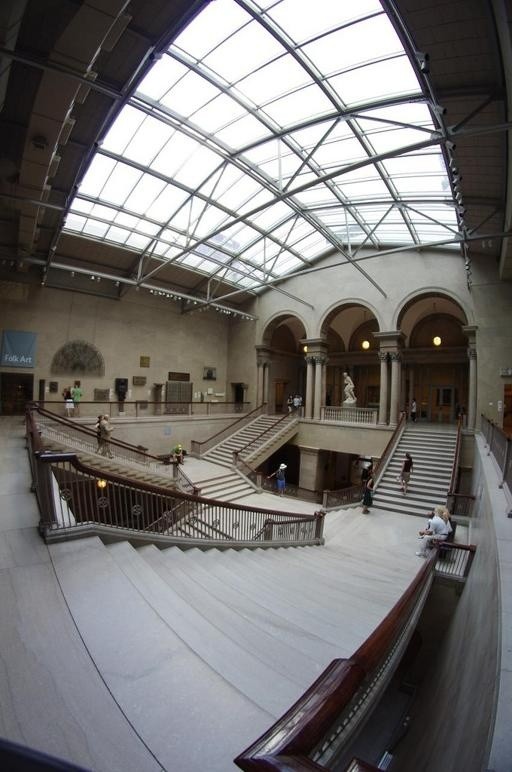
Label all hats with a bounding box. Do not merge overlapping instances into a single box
[280,464,287,469]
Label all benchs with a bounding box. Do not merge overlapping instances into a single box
[426,518,457,561]
[158,450,187,465]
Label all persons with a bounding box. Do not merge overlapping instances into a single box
[173,444,184,465]
[411,398,417,422]
[295,393,302,405]
[72,384,83,416]
[361,469,376,514]
[63,388,73,415]
[100,415,116,458]
[287,393,293,416]
[418,506,452,538]
[415,511,446,556]
[399,452,413,495]
[343,371,356,402]
[95,414,104,455]
[267,463,287,497]
[292,395,300,408]
